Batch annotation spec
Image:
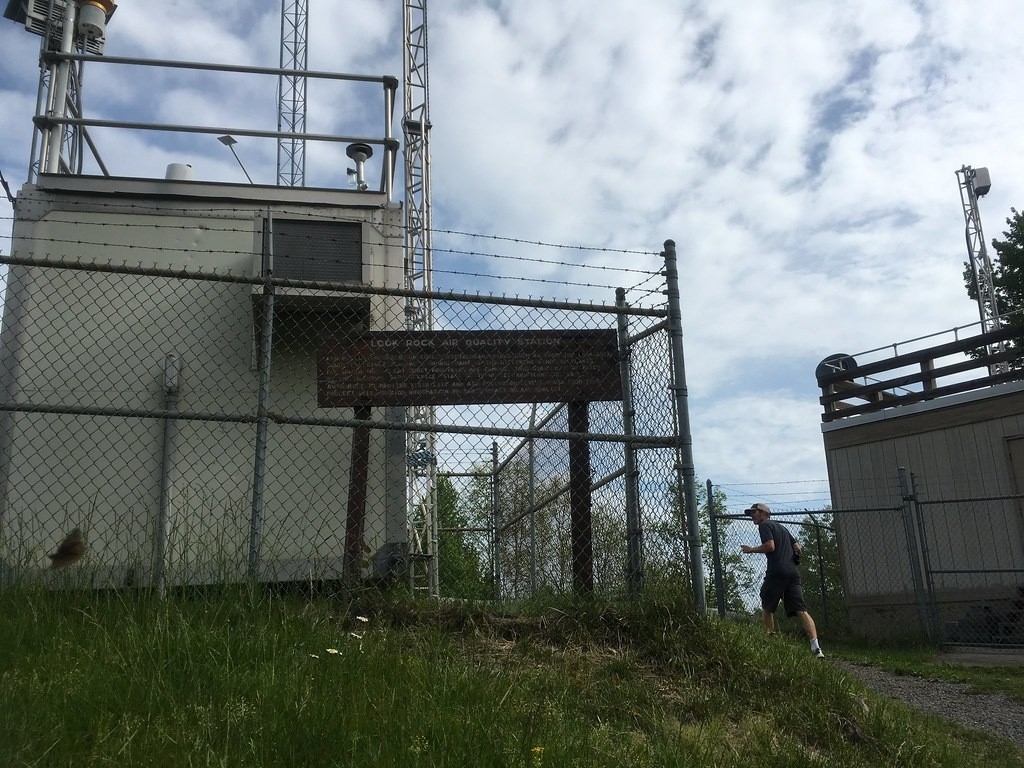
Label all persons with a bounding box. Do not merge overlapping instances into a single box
[740,503,825,660]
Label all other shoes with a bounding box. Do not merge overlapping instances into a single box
[812,647,825,657]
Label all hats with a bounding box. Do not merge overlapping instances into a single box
[744,503,771,517]
